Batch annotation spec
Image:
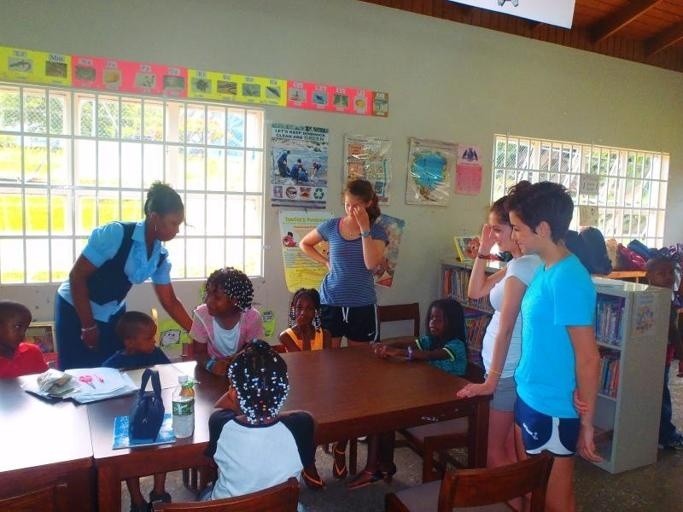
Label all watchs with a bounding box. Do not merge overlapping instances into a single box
[359,230,371,237]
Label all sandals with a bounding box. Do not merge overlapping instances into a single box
[301,469,326,491]
[332,444,348,480]
[377,472,392,483]
[346,470,383,490]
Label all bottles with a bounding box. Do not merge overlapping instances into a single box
[169,374,196,440]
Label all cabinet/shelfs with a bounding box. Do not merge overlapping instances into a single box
[441,255,671,476]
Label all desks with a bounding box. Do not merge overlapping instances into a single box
[0,373,92,508]
[84,343,492,507]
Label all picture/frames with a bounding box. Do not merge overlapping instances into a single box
[23,322,56,354]
[453,235,480,261]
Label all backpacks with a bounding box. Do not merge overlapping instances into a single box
[575,227,613,275]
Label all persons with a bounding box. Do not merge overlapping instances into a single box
[277,149,309,183]
[195,339,316,512]
[299,179,387,350]
[345,298,469,489]
[645,255,683,451]
[504,182,604,511]
[99,311,172,512]
[279,288,348,493]
[188,268,264,375]
[54,181,194,373]
[456,195,543,512]
[0,301,50,381]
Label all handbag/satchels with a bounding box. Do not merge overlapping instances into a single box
[129,369,165,440]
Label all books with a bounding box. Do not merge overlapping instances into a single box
[112,414,176,450]
[443,268,496,313]
[593,297,625,347]
[462,307,493,363]
[454,235,482,264]
[599,351,620,399]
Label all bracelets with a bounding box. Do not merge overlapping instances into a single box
[407,346,413,361]
[80,325,97,332]
[488,369,502,375]
[477,253,491,259]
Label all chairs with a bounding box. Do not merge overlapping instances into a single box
[384,450,555,511]
[395,362,485,483]
[154,478,298,512]
[376,298,422,349]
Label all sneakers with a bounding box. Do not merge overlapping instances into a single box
[131,505,149,512]
[150,488,170,506]
[657,431,683,449]
